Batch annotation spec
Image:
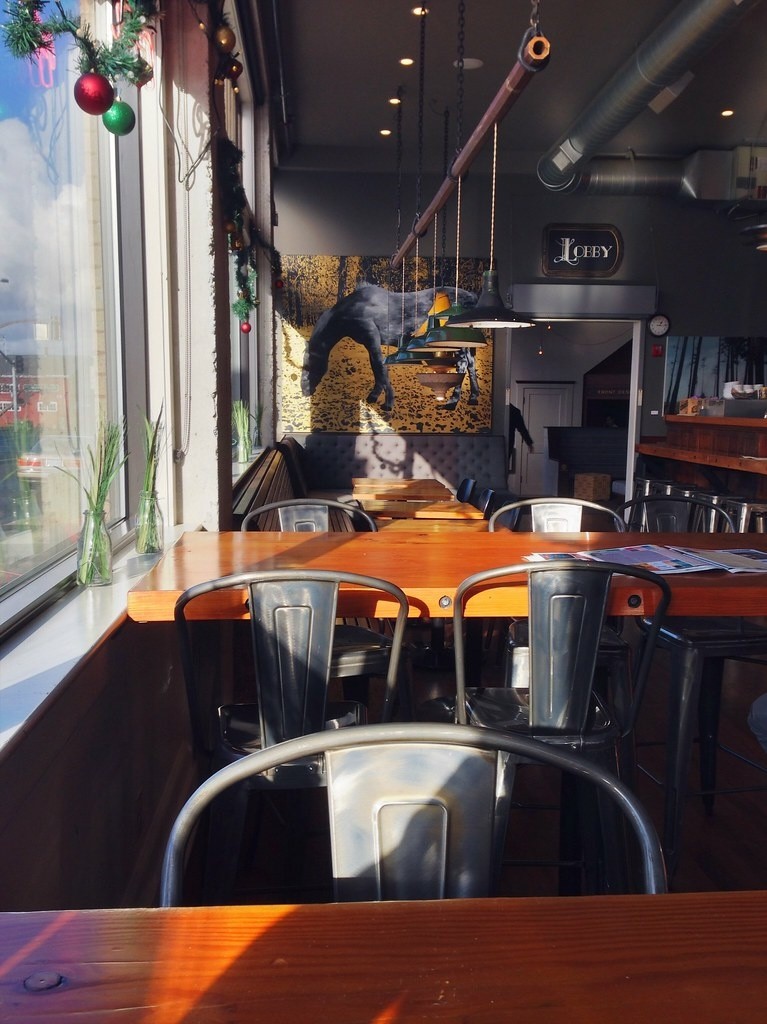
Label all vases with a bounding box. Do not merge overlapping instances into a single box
[134,491,163,555]
[77,509,112,587]
[238,438,252,463]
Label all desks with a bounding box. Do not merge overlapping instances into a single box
[125,479,767,622]
[0,890,767,1024]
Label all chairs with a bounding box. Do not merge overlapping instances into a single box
[160,478,767,909]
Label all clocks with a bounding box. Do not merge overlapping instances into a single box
[648,314,670,338]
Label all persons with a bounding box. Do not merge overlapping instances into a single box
[509,403,533,462]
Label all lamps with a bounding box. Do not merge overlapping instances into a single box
[384,122,536,365]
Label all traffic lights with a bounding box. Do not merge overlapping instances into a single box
[14,355,24,373]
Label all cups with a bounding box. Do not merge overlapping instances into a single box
[754,384,763,392]
[743,385,753,391]
[733,384,742,392]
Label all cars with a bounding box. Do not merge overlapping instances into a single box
[15,431,99,490]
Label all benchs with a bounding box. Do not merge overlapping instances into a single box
[232,449,357,532]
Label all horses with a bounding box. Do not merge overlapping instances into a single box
[301,282,481,410]
